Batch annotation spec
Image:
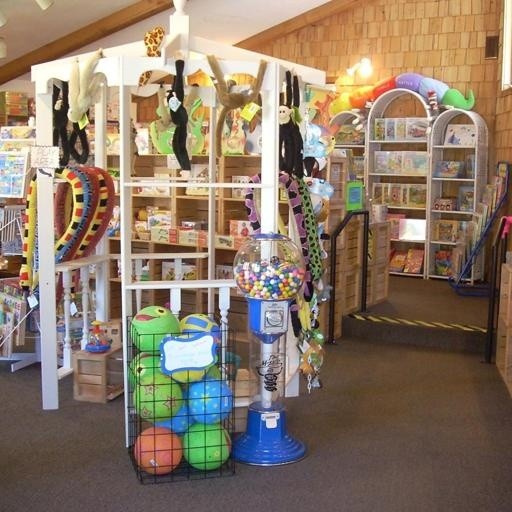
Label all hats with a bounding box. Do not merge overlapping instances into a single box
[317,124,336,156]
[302,123,326,158]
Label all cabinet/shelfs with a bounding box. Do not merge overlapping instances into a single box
[0,127,66,371]
[450,162,509,294]
[32,36,326,408]
[330,89,489,283]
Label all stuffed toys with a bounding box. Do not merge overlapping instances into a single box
[156,82,173,132]
[302,124,335,259]
[183,84,199,132]
[279,70,304,179]
[244,171,325,381]
[52,80,69,174]
[67,48,108,122]
[207,54,266,158]
[248,94,263,132]
[20,165,115,312]
[166,59,191,180]
[329,73,474,116]
[69,109,89,164]
[139,27,165,87]
